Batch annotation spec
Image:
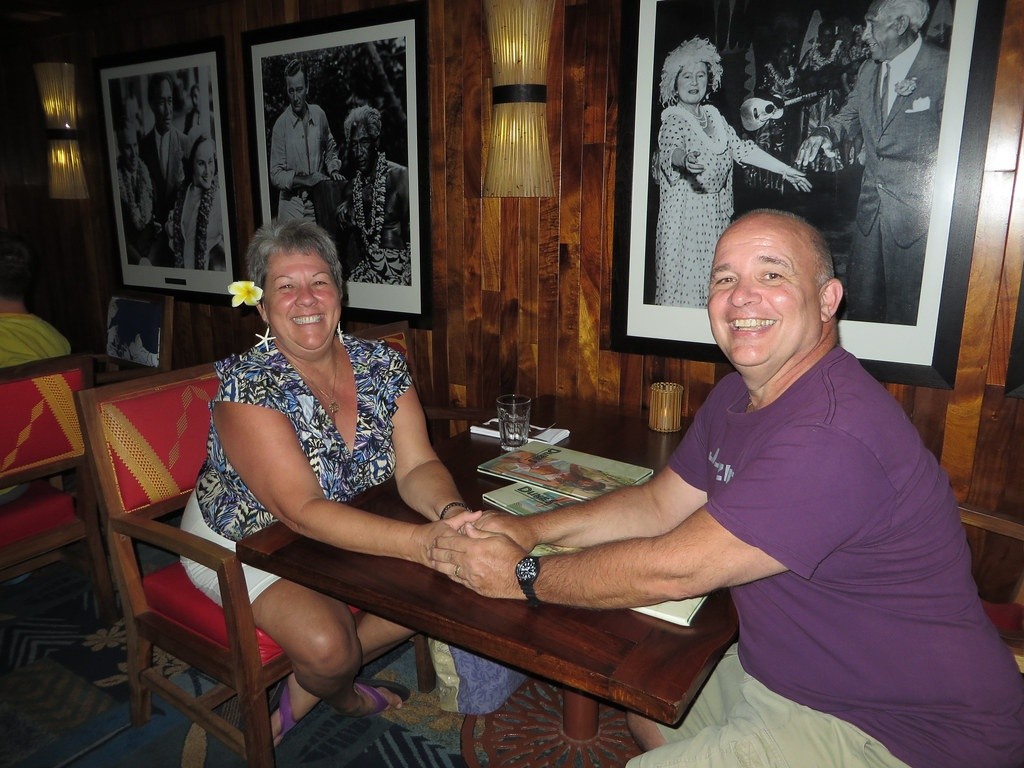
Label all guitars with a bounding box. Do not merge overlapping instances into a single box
[738,86,830,133]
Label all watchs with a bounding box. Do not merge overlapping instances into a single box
[515,555,541,603]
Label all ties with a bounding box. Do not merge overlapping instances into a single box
[880,62,892,129]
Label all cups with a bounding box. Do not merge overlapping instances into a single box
[496,394,536,452]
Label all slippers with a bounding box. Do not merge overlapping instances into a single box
[270,676,298,750]
[353,680,410,717]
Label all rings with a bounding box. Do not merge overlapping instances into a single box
[454,565,461,577]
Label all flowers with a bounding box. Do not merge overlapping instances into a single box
[228,280,263,307]
[895,79,916,97]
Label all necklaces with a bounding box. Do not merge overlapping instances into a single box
[273,342,340,414]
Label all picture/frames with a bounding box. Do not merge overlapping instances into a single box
[89,35,240,306]
[611,0,1007,390]
[240,0,435,331]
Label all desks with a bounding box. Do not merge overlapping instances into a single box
[237,394,741,768]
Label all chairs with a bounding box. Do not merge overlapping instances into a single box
[0,292,494,768]
[958,501,1024,675]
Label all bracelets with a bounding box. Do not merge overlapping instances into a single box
[440,501,471,520]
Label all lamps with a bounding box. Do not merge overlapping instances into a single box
[33,60,90,199]
[483,0,557,198]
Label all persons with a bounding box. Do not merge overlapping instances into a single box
[798,0,953,325]
[180,215,483,748]
[269,59,341,221]
[337,105,410,285]
[116,74,225,272]
[1,232,72,586]
[428,209,1024,768]
[750,20,872,190]
[649,36,812,309]
